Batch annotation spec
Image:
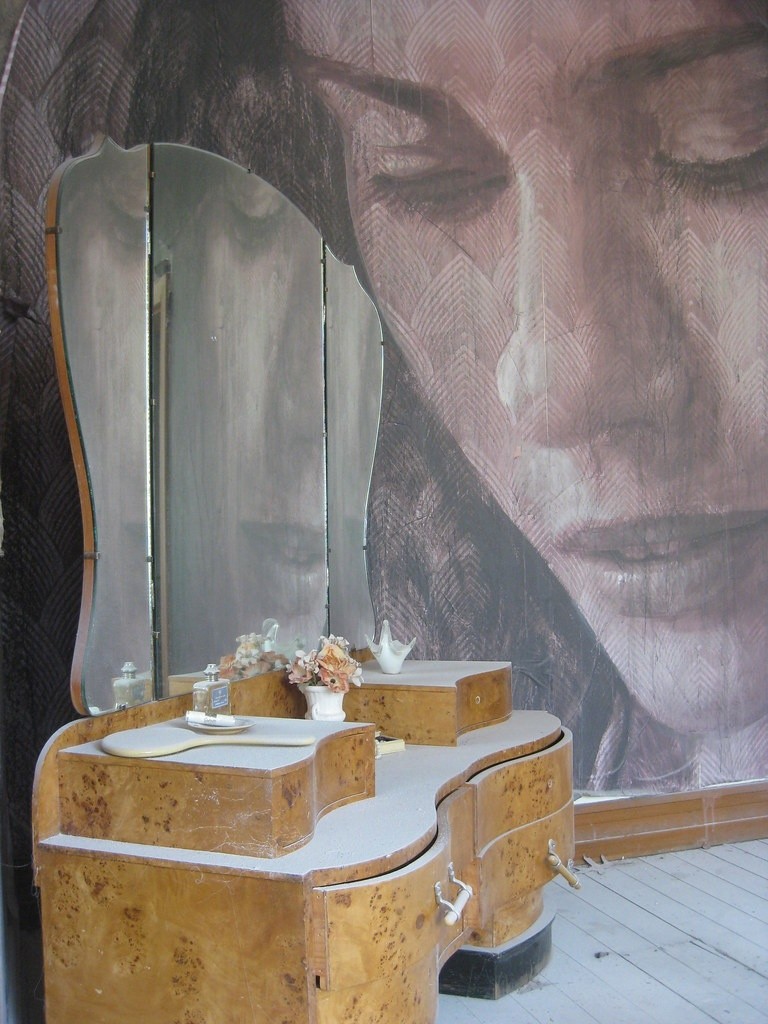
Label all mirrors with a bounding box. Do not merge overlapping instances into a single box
[47,134,388,718]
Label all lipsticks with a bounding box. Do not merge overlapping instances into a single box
[182,708,236,727]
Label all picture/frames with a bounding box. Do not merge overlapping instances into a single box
[193,164,332,646]
[0,1,768,856]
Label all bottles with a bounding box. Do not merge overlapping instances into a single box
[193,664,231,717]
[112,662,146,711]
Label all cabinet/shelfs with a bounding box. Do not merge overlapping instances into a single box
[26,645,581,1024]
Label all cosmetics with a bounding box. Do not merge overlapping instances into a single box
[187,663,236,715]
[110,661,145,707]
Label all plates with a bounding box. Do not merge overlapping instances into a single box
[188,718,255,735]
